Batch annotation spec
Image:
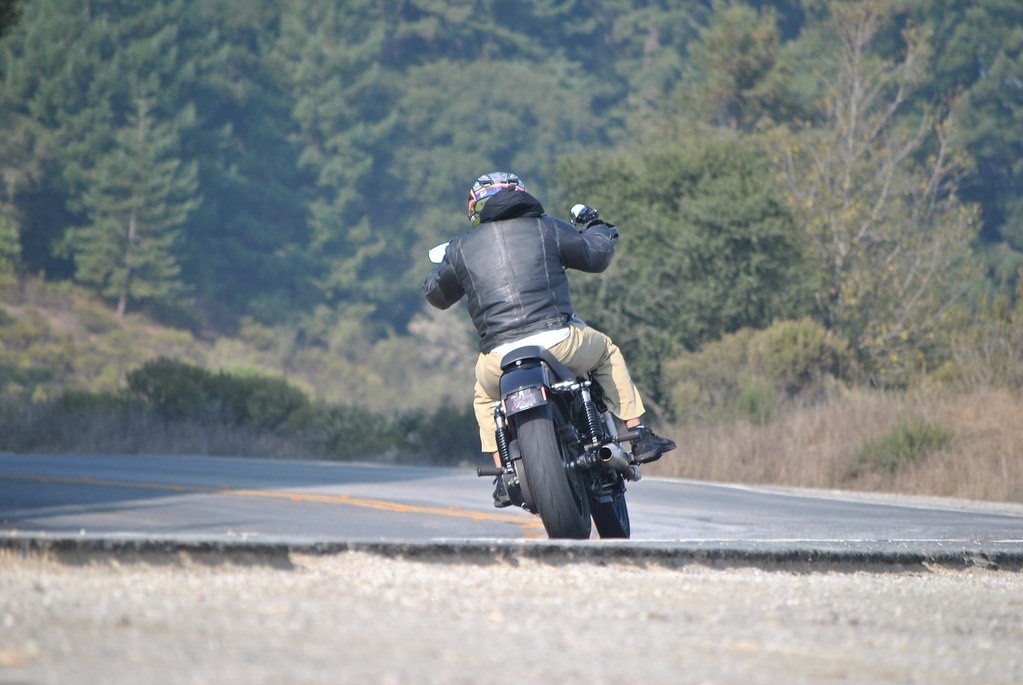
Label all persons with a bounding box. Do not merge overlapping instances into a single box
[425,171,677,508]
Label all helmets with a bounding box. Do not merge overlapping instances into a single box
[468,171,528,229]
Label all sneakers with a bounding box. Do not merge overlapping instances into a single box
[492,475,513,507]
[628,424,677,463]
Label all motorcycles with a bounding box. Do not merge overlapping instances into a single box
[428,203,663,538]
